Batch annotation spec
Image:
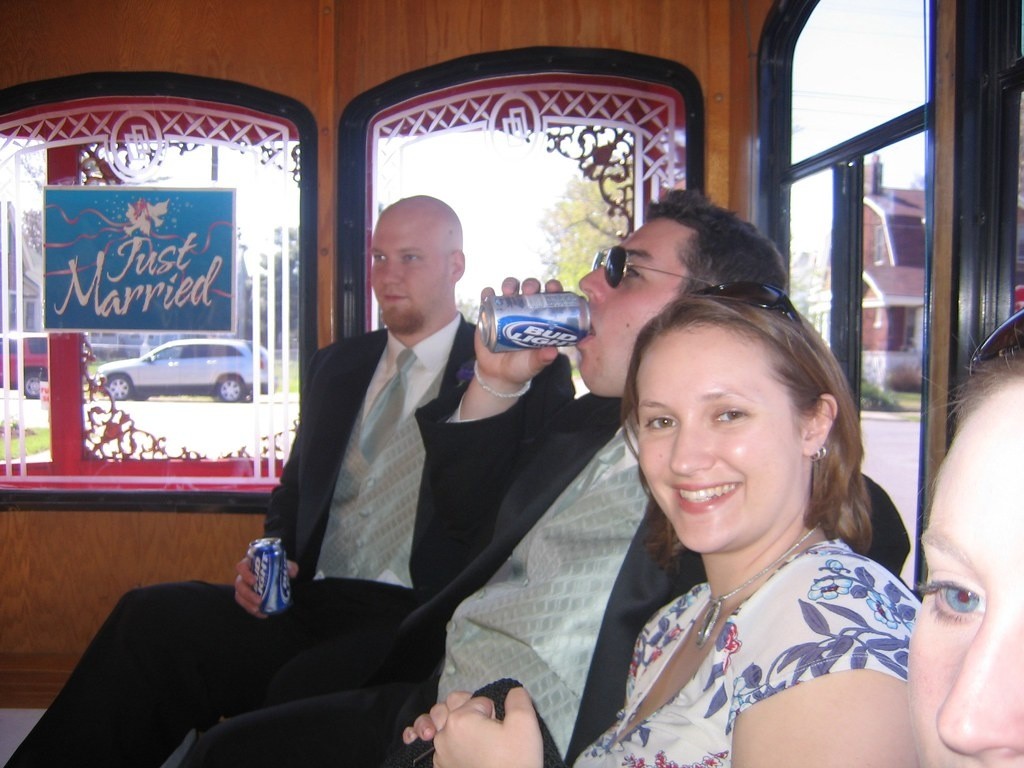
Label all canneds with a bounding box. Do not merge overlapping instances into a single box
[246,537,291,615]
[479,292,592,352]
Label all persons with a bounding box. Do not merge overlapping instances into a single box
[402,286,923,767]
[3,192,576,768]
[179,189,913,768]
[907,329,1024,768]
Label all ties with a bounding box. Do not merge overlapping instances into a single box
[582,429,626,494]
[361,348,414,465]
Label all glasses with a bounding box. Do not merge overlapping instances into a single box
[593,245,722,288]
[692,280,801,324]
[969,307,1023,377]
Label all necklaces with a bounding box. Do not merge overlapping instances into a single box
[697,521,823,647]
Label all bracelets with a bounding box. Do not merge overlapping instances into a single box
[474,358,533,399]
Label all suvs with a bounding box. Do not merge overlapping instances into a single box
[94,337,274,401]
[1,334,52,401]
